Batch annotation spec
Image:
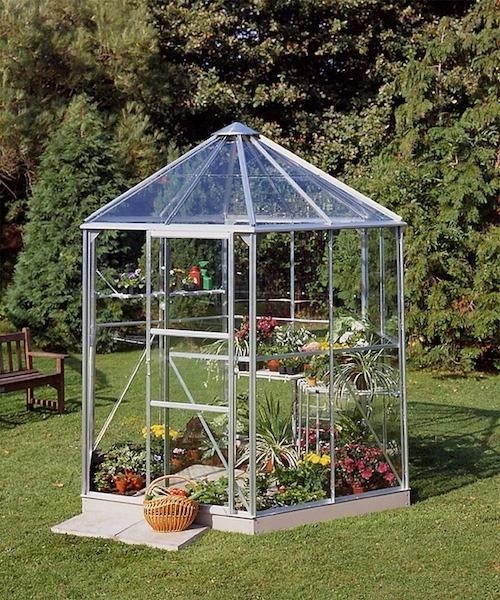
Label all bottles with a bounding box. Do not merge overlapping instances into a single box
[188,264,201,289]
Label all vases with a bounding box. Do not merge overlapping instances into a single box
[117,284,199,295]
[237,360,317,385]
[353,486,366,494]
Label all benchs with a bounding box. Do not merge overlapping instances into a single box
[0,326,70,415]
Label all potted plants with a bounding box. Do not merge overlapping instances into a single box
[92,439,157,496]
[320,335,399,402]
[182,388,299,472]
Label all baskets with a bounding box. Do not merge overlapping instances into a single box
[143,475,199,532]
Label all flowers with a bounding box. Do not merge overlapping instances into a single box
[296,422,398,488]
[232,311,370,364]
[114,265,198,289]
[141,423,179,454]
